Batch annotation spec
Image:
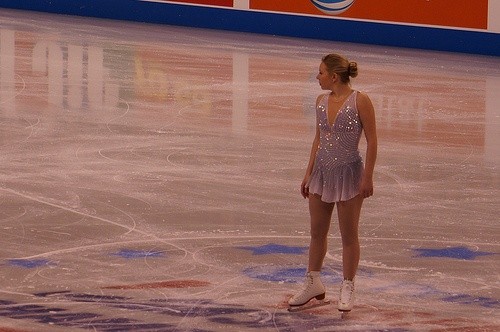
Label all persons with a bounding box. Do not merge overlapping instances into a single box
[287,54,379,318]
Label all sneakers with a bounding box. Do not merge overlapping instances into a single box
[336,280,355,318]
[287,271,331,313]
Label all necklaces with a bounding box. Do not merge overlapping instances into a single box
[334,89,352,103]
